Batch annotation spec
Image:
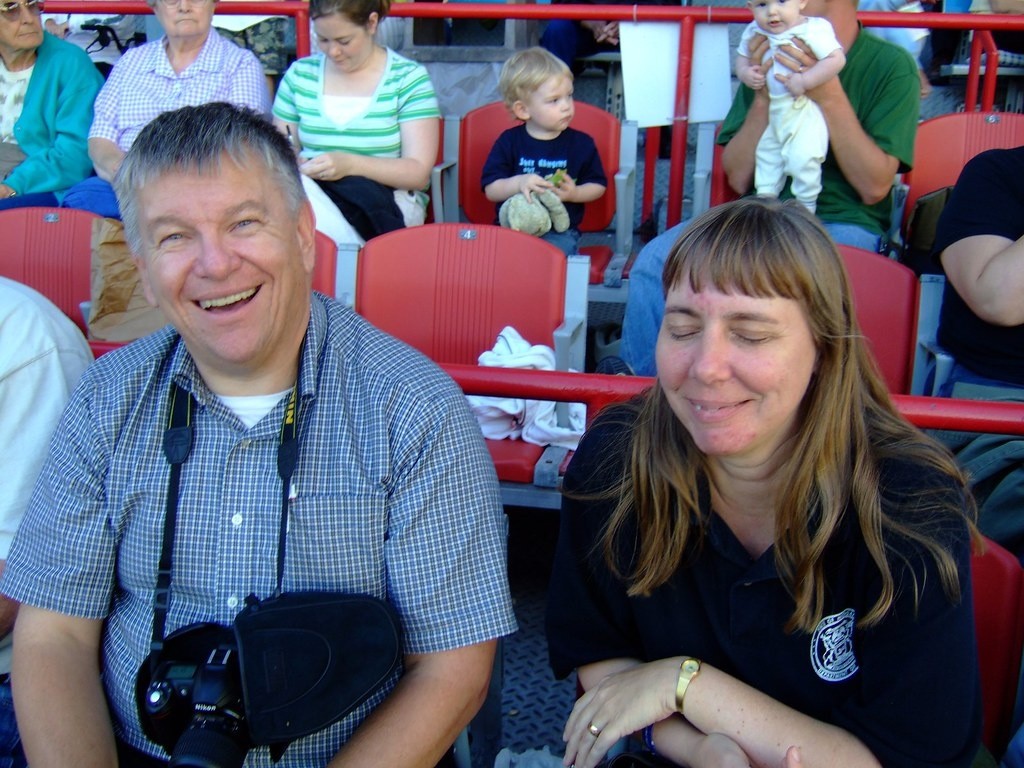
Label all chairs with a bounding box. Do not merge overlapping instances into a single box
[0,101,1024,759]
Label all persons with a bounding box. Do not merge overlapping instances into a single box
[0,0,441,244]
[0,101,519,768]
[546,194,986,768]
[538,0,683,73]
[480,45,607,257]
[595,1,921,376]
[928,143,1024,569]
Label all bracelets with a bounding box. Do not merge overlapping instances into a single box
[642,723,656,755]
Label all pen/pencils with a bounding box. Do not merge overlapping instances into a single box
[286,125,296,146]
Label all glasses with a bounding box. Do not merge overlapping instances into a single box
[0,0,45,22]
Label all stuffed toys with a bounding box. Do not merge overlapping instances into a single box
[499,189,570,237]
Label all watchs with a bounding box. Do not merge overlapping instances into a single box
[676,657,702,715]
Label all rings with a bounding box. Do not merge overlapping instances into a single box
[318,172,324,177]
[587,721,601,737]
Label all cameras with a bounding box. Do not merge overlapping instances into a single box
[135,622,258,768]
